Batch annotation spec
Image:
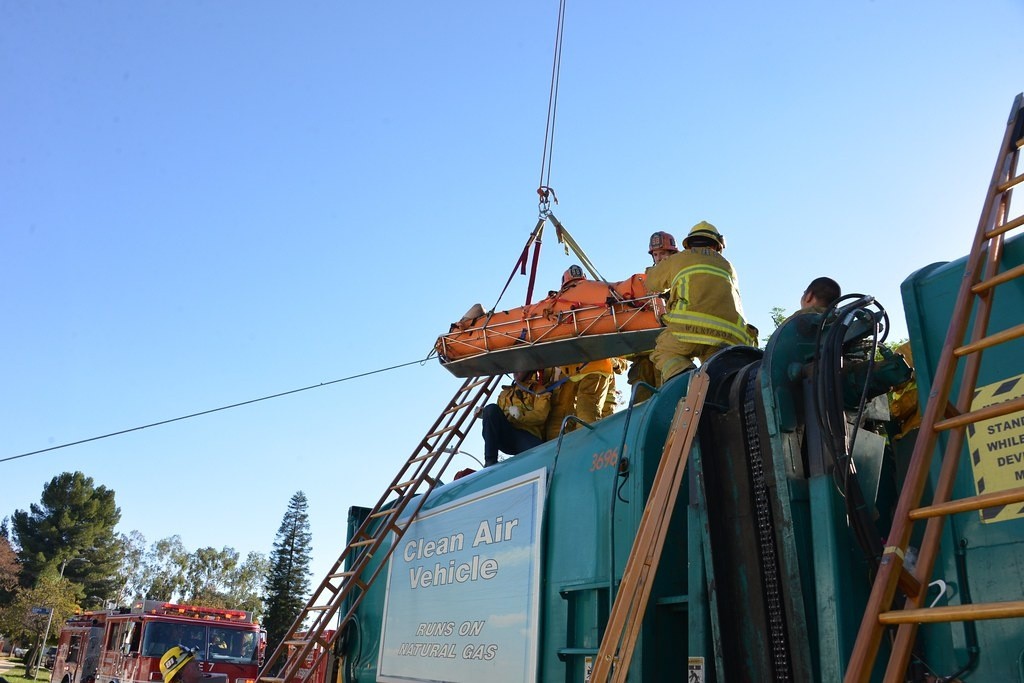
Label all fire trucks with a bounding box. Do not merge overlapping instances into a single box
[50,598,267,683]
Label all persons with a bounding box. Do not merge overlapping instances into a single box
[641,220,758,380]
[160,643,203,683]
[482,369,553,468]
[801,276,841,311]
[547,267,663,431]
[648,231,678,265]
[209,633,227,648]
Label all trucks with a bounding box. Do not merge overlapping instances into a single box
[284,630,338,683]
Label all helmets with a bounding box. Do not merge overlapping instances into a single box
[560,264,587,288]
[648,230,678,254]
[158,645,196,683]
[682,220,725,247]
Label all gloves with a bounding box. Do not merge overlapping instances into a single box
[509,406,521,420]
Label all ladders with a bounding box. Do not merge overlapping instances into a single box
[840,95,1024,682]
[252,375,501,682]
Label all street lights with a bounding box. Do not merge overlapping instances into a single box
[33,557,91,681]
